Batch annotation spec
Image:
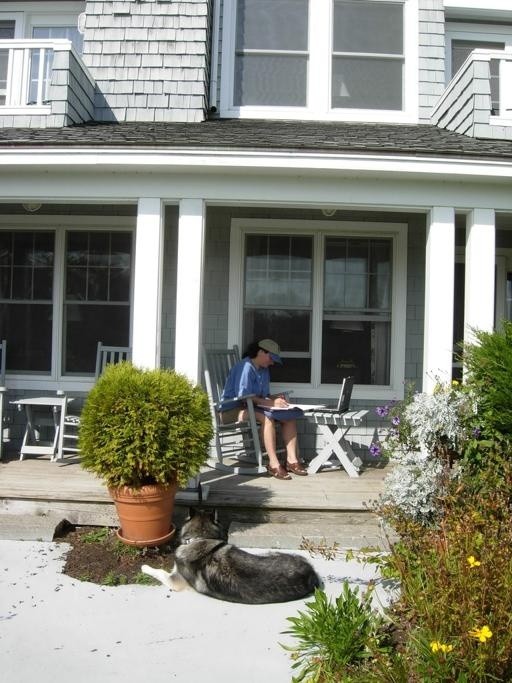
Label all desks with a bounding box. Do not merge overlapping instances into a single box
[305,406,373,478]
[9,394,74,462]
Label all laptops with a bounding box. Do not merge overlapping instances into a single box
[310,376,355,414]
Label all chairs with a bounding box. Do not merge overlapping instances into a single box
[201,343,300,477]
[60,338,132,462]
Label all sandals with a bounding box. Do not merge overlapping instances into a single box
[267,464,292,480]
[284,461,308,476]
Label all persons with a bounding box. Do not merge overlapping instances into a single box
[218,337,308,481]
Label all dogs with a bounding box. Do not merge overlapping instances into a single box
[141,503,319,604]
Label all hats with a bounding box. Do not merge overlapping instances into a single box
[259,339,283,365]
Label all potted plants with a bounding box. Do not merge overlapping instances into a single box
[78,357,219,553]
